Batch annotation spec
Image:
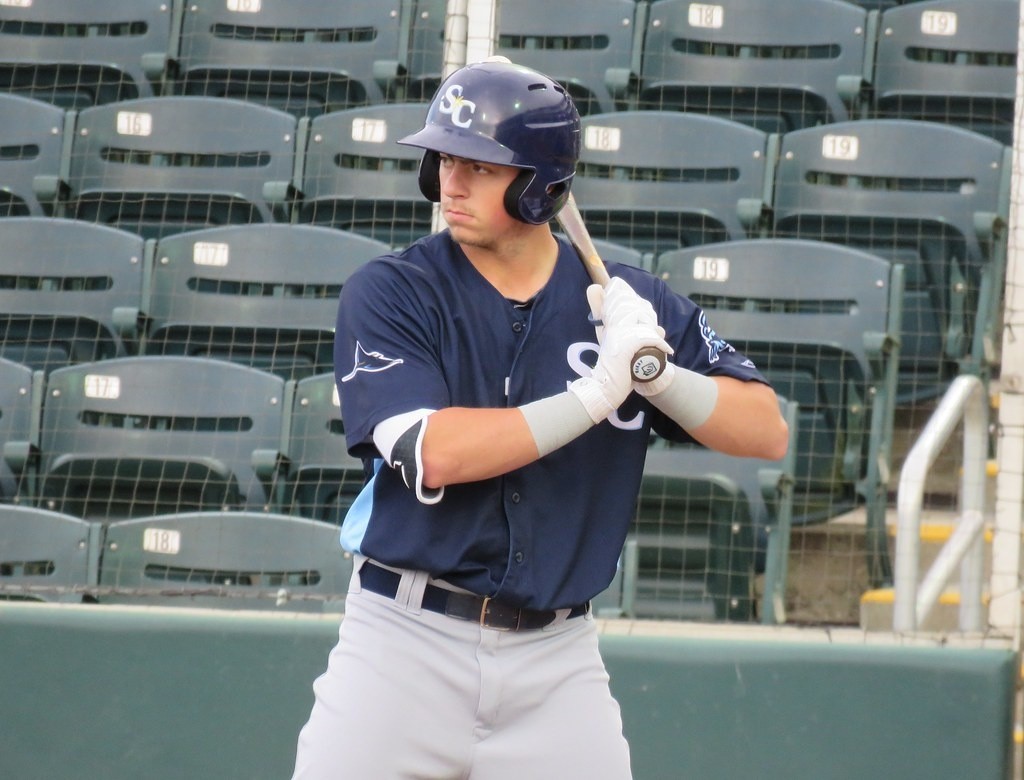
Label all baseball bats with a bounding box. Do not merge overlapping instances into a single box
[478,54,669,385]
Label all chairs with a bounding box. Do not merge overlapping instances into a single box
[0,0,1024,622]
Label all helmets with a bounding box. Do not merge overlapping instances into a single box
[396,62,582,225]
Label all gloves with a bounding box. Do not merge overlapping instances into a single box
[567,276,677,424]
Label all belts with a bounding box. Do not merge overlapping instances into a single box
[358,562,589,632]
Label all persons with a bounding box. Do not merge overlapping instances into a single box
[290,64,790,780]
